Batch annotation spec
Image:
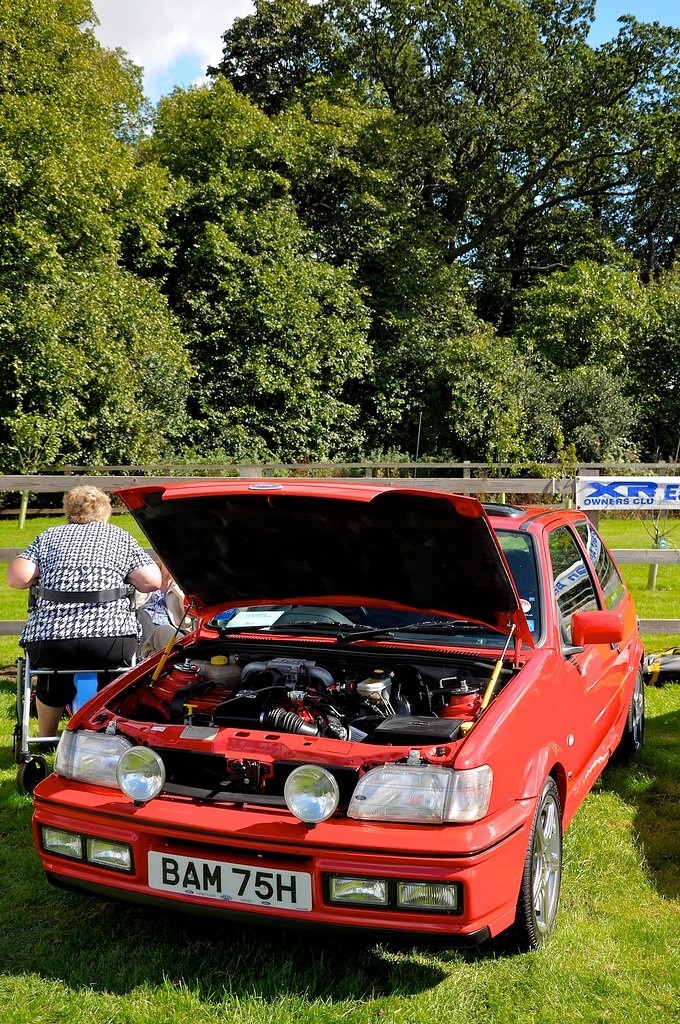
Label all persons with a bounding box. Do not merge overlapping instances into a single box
[6,484,163,749]
[136,559,194,663]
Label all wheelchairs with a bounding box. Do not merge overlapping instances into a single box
[11,630,142,796]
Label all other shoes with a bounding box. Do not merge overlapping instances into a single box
[36,742,54,753]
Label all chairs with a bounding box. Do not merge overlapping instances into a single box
[503,549,537,596]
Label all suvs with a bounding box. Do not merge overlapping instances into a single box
[28,477,649,951]
